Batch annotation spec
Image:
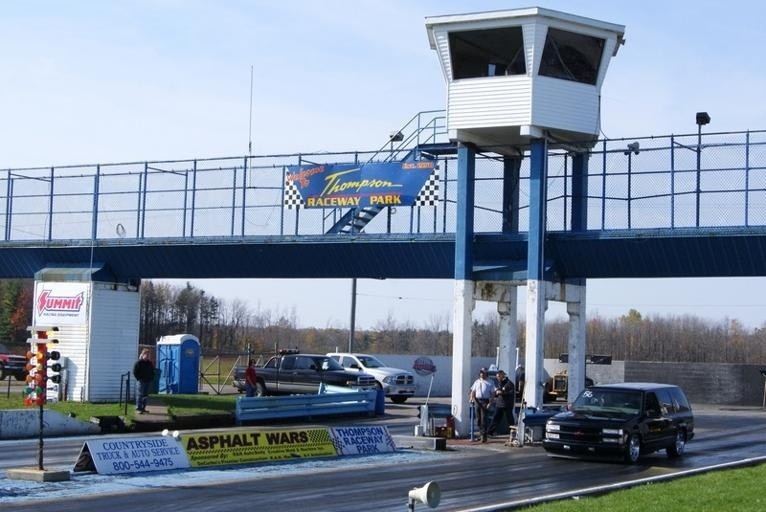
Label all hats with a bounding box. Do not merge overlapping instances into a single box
[479,367,487,374]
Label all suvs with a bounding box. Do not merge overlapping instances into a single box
[542,382,697,464]
[0,345,28,381]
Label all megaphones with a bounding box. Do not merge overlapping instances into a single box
[409,480,440,509]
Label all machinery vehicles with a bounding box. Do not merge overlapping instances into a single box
[543,368,595,404]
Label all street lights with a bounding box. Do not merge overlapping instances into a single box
[386,131,404,233]
[695,110,712,226]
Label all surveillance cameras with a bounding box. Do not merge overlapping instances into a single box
[634,142,639,155]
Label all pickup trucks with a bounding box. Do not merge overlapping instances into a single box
[320,351,417,404]
[233,347,375,395]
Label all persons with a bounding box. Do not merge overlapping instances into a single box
[245,359,257,397]
[132,346,154,414]
[487,370,515,435]
[469,368,495,443]
